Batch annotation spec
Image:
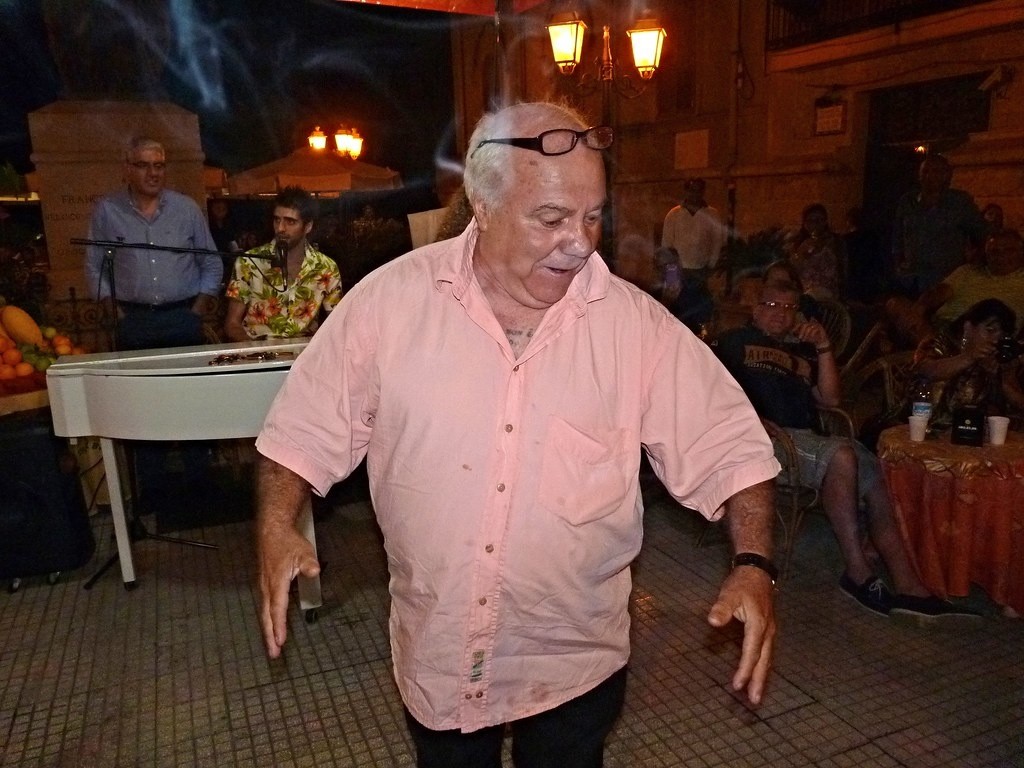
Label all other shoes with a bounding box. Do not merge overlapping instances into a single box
[136,483,165,516]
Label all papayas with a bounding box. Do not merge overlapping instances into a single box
[0,305,42,346]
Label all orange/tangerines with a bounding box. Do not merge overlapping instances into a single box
[0,327,73,381]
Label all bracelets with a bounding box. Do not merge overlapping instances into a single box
[732,552,778,583]
[815,344,831,354]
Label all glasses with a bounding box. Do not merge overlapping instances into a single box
[759,300,800,312]
[128,160,166,170]
[470,123,614,159]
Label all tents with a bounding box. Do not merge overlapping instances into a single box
[25,164,228,189]
[226,139,405,199]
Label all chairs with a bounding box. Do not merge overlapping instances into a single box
[812,298,852,361]
[851,348,918,426]
[691,403,857,591]
[843,320,884,376]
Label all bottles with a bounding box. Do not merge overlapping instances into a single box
[911,376,938,439]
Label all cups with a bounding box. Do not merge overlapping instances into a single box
[908,416,929,442]
[988,416,1010,445]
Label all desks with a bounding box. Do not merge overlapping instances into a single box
[0,388,50,417]
[877,422,1024,616]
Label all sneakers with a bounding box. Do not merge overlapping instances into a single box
[837,569,895,617]
[889,592,983,630]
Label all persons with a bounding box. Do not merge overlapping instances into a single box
[79,136,225,510]
[615,150,1024,619]
[0,197,260,315]
[225,184,342,345]
[252,100,783,768]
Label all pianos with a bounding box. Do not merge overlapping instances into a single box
[45,335,323,625]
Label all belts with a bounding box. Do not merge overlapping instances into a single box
[118,294,200,314]
